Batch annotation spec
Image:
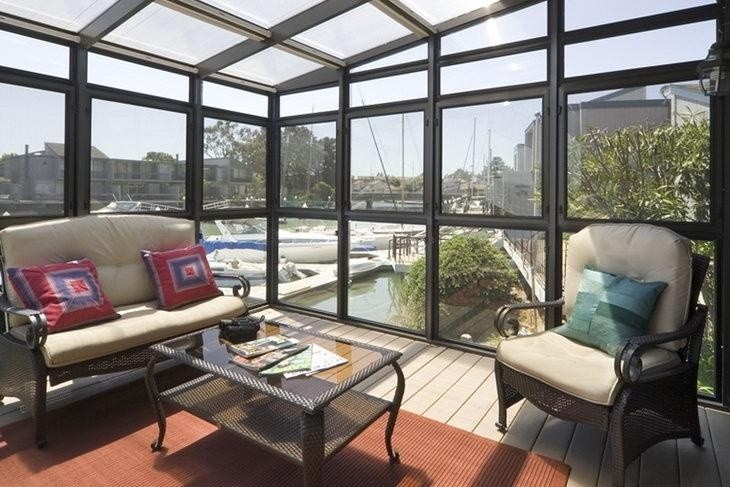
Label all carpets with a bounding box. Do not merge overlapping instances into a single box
[0,351,571,486]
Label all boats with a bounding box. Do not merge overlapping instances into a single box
[207,234,338,287]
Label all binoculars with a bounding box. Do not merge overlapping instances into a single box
[218,316,268,342]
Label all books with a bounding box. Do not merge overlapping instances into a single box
[227,334,349,380]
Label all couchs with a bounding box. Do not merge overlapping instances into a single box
[0,213,253,449]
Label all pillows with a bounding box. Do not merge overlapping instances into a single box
[140,241,223,311]
[550,262,668,361]
[8,256,122,337]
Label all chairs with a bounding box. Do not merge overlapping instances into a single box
[493,224,710,487]
